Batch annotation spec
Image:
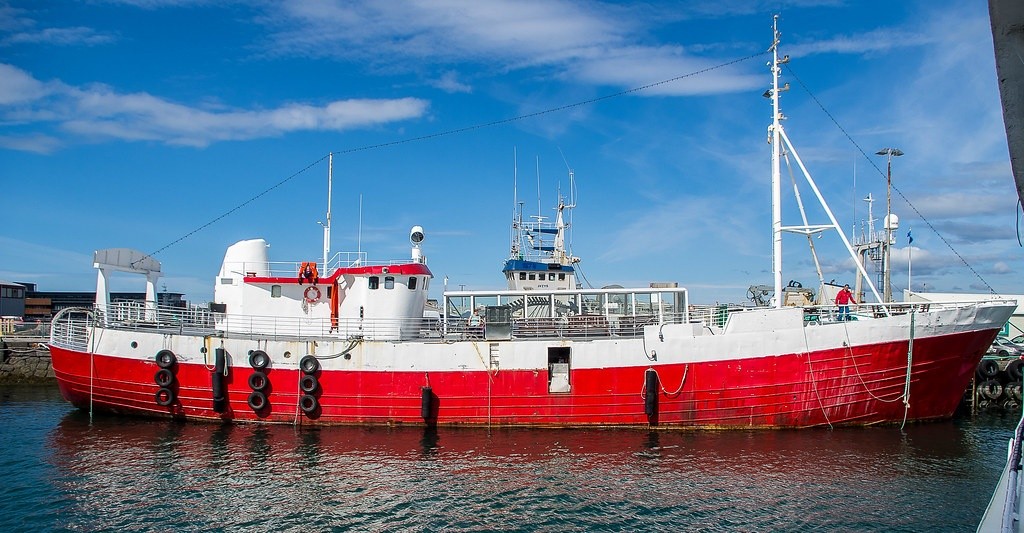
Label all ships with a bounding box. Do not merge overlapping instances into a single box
[47,12,1020,432]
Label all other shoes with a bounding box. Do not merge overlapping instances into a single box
[468,337,472,341]
[476,337,479,341]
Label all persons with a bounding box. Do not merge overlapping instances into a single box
[835,284,857,321]
[510,246,518,258]
[468,309,481,341]
[33,320,43,335]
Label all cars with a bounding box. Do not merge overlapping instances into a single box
[1009,332,1024,354]
[994,336,1022,356]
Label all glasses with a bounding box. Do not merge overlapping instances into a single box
[845,286,849,289]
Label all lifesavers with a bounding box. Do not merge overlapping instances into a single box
[247,350,268,409]
[154,348,175,405]
[303,286,322,303]
[298,356,319,413]
